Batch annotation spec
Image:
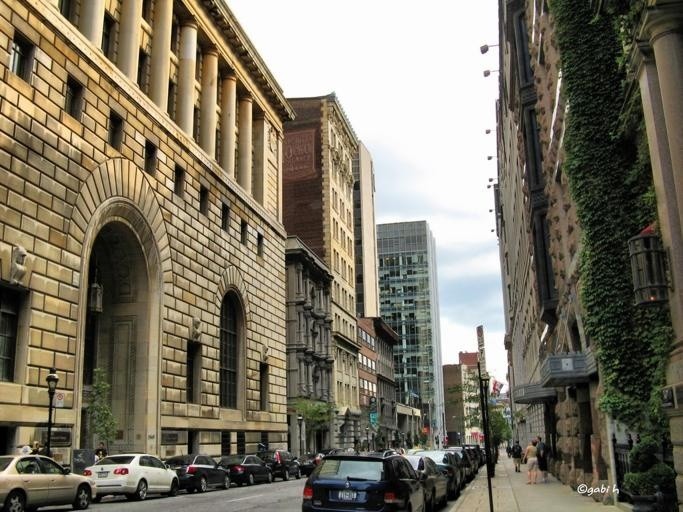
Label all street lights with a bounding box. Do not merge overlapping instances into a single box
[401,430,406,447]
[452,416,460,447]
[297,414,303,456]
[44,366,60,456]
[365,426,370,452]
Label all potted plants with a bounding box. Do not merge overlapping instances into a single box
[622,436,676,512]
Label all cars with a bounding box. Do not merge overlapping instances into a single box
[84,448,327,504]
[0,451,98,511]
[330,444,488,512]
[299,444,429,512]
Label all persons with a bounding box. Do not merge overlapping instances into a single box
[95,442,107,462]
[256,441,266,460]
[506,436,549,484]
[21,440,53,473]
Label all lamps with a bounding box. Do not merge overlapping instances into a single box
[88,250,104,312]
